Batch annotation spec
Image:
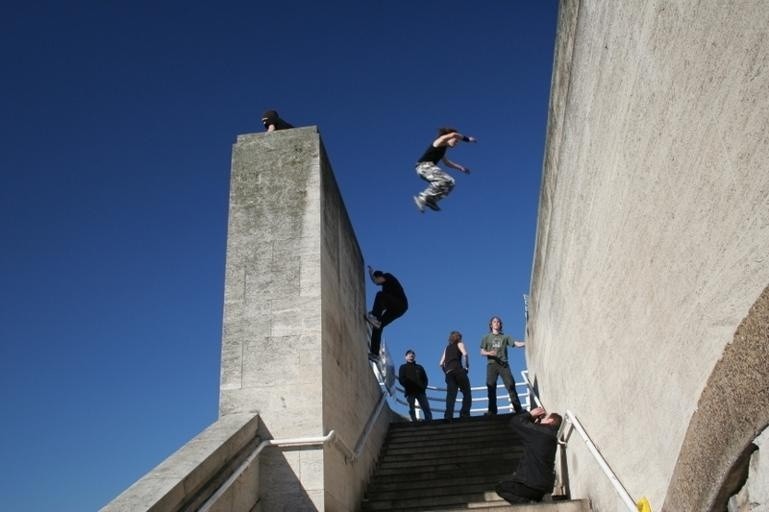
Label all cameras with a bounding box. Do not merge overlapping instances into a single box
[538,407,545,419]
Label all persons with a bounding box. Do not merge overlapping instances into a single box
[440,331,473,420]
[479,316,528,417]
[398,348,433,422]
[363,264,408,361]
[261,109,296,131]
[494,406,564,506]
[412,126,477,214]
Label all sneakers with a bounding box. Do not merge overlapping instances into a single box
[368,352,379,364]
[426,198,440,211]
[364,315,382,328]
[415,195,425,212]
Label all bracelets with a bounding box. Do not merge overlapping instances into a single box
[463,135,470,142]
[465,367,469,370]
